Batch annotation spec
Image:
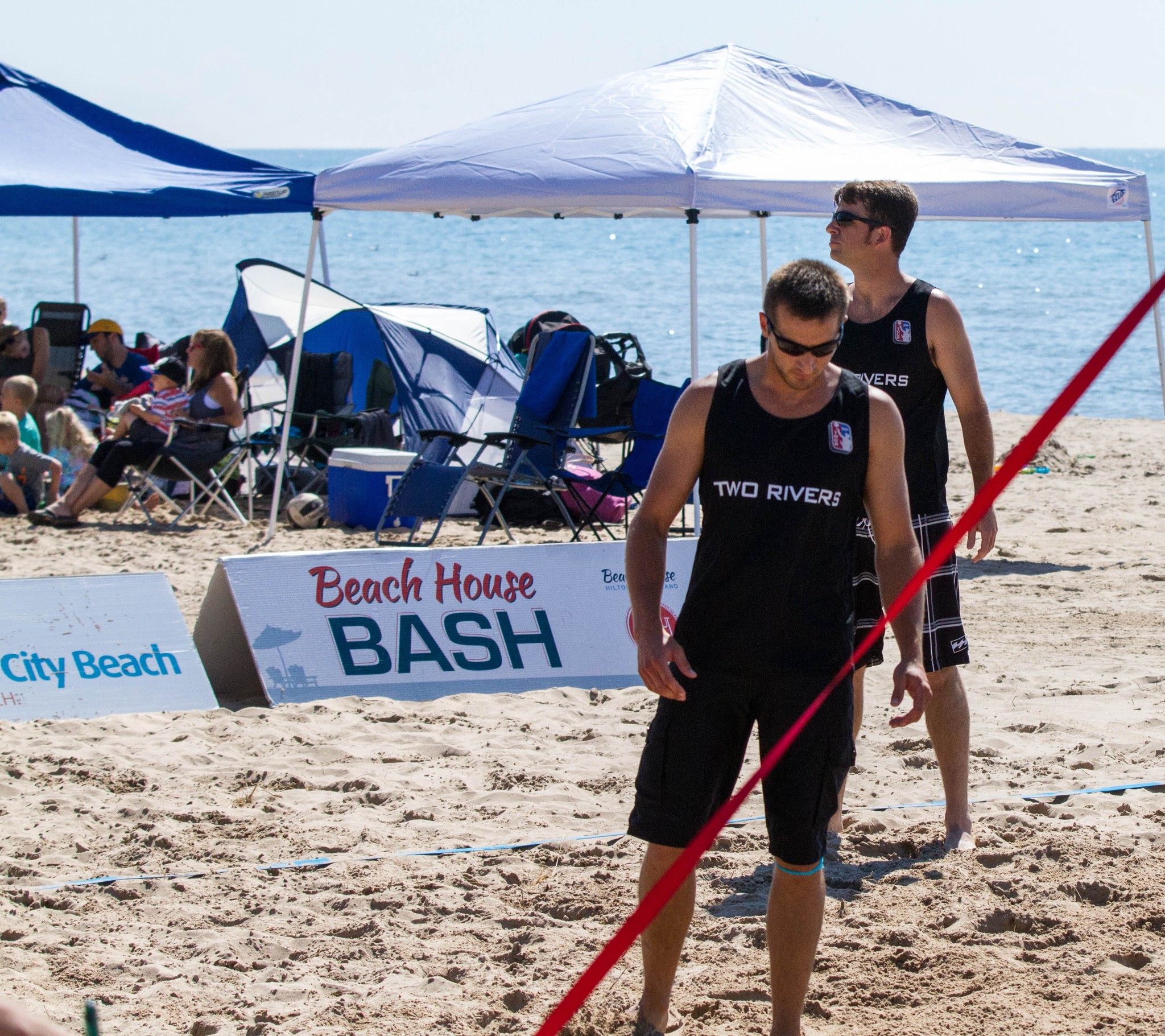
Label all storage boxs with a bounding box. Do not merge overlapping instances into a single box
[323,442,424,533]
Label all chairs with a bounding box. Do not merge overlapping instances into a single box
[29,295,93,398]
[112,323,731,546]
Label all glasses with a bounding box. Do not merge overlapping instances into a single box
[189,341,201,349]
[767,320,843,358]
[830,211,880,227]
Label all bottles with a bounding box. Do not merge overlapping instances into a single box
[1020,466,1050,473]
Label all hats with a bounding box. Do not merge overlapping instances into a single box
[139,357,188,385]
[81,320,123,339]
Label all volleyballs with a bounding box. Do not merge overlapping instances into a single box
[285,493,327,529]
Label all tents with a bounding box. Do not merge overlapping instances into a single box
[267,42,1165,543]
[223,256,603,521]
[0,60,338,301]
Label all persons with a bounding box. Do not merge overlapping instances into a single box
[817,182,998,853]
[623,260,933,1036]
[0,296,244,523]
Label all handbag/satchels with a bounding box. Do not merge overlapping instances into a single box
[473,478,571,525]
[556,452,632,521]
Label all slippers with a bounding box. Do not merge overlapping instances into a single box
[27,507,82,527]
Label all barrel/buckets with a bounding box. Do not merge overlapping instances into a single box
[99,481,129,512]
